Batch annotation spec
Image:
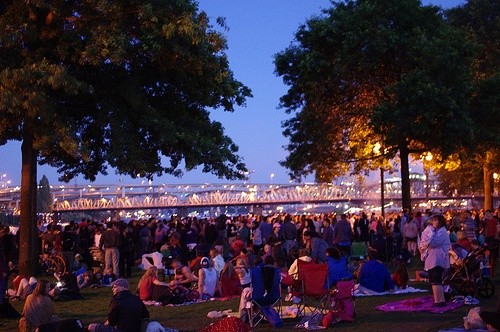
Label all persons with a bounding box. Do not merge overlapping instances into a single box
[89,279,151,332]
[418,215,452,307]
[0,209,500,300]
[21,279,58,327]
[252,256,282,305]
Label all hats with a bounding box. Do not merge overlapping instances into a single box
[200,257,210,265]
[111,279,129,288]
[273,223,281,228]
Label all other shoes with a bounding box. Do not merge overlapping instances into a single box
[432,302,448,307]
[10,296,20,302]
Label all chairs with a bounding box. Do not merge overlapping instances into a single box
[245,258,357,332]
